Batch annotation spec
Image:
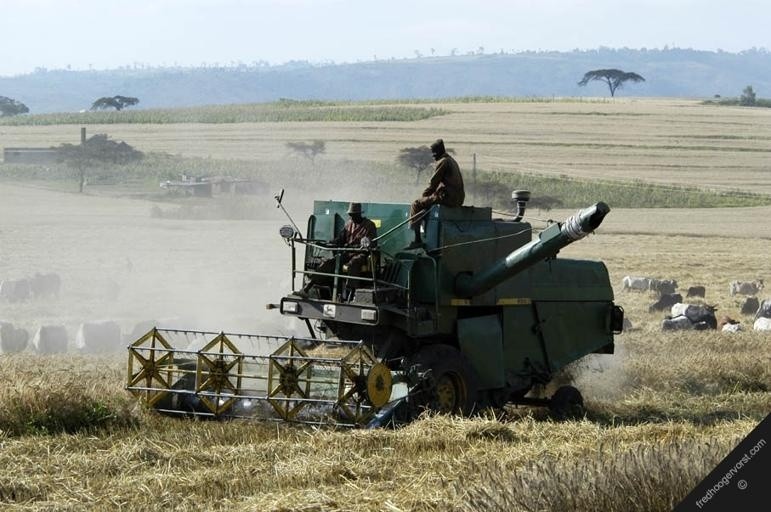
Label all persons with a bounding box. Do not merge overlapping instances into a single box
[403,138,465,251]
[322,202,380,301]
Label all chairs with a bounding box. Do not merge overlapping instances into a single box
[341,247,381,299]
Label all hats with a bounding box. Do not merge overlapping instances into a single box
[345,201,364,215]
[430,138,446,151]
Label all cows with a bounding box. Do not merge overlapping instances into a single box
[621,275,771,334]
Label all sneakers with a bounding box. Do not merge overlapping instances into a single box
[404,240,424,250]
